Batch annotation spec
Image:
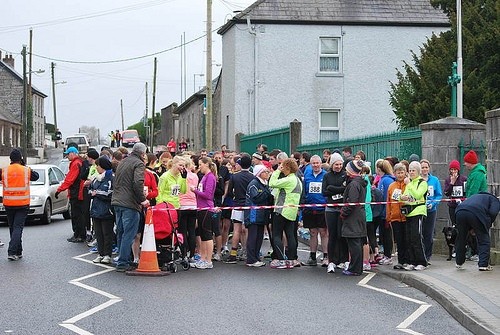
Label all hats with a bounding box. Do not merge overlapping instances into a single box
[64,147,79,154]
[252,152,262,160]
[329,153,363,177]
[87,150,99,159]
[449,160,461,171]
[100,157,112,170]
[464,149,478,164]
[253,165,264,178]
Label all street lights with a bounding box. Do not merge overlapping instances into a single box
[23,69,46,166]
[52,81,66,132]
[194,74,205,93]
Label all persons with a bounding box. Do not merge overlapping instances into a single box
[83,137,442,275]
[463,150,487,262]
[109,131,116,147]
[55,147,90,242]
[116,130,121,147]
[443,159,466,258]
[456,192,500,271]
[55,129,62,140]
[0,149,39,260]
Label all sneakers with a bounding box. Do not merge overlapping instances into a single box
[101,255,112,264]
[190,259,213,269]
[93,255,103,263]
[394,263,425,270]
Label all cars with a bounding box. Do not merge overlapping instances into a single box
[58,133,93,177]
[0,165,71,225]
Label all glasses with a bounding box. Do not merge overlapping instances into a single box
[215,157,222,160]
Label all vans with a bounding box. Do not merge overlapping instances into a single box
[121,130,141,148]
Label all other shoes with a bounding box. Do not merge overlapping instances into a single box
[326,262,358,276]
[225,253,247,263]
[112,247,118,254]
[87,239,97,247]
[269,258,329,268]
[363,255,392,271]
[246,261,265,267]
[479,265,493,271]
[67,237,84,242]
[91,248,98,252]
[8,254,22,261]
[214,254,223,261]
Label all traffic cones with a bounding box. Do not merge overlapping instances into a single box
[125,206,170,276]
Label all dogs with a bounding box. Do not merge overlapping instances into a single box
[442,227,477,263]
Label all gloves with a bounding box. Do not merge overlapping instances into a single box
[180,168,187,178]
[55,192,60,199]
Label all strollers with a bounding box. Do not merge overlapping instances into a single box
[144,200,190,273]
